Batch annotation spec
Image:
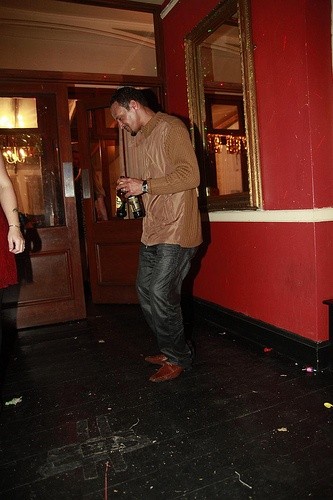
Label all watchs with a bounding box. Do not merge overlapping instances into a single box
[141,181,147,193]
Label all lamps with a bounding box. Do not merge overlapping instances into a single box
[2,148,33,164]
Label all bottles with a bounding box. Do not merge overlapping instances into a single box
[125,176,145,219]
[116,175,127,218]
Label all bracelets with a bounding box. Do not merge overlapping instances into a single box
[8,225,18,228]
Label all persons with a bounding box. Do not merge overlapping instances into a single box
[110,86,207,383]
[0,152,28,408]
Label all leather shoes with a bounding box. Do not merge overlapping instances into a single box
[147,360,191,384]
[145,350,170,365]
